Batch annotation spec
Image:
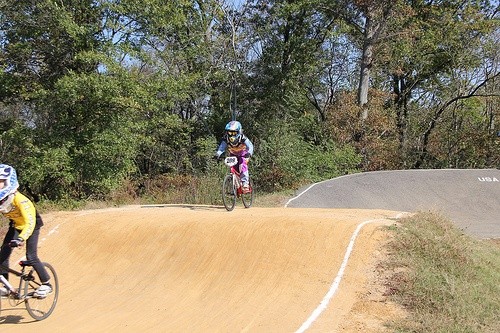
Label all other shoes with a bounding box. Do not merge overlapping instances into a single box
[244,185,250,193]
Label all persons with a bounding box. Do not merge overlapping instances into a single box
[0,164,53,297]
[213,121,253,199]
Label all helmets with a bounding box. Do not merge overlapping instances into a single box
[225,120,242,144]
[0,164,19,200]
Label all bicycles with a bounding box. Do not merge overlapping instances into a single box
[211,154,254,211]
[0,238,59,321]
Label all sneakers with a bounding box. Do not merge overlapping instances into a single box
[0,287,11,295]
[33,283,52,297]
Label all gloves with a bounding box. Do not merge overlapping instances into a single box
[9,238,23,247]
[245,153,250,158]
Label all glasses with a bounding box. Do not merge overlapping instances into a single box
[228,131,237,136]
